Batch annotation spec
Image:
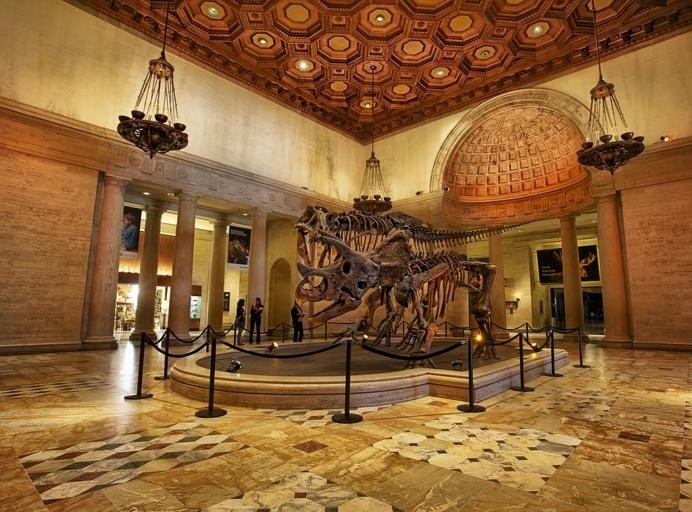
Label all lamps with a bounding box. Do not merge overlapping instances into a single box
[266,341,281,352]
[225,359,241,374]
[352,65,392,215]
[361,333,369,343]
[117,0,188,160]
[576,1,645,175]
[451,358,465,373]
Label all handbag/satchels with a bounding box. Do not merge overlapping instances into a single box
[234,317,240,326]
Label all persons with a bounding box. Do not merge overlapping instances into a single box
[233,298,247,346]
[290,299,305,343]
[121,212,139,250]
[249,297,264,344]
[228,237,248,264]
